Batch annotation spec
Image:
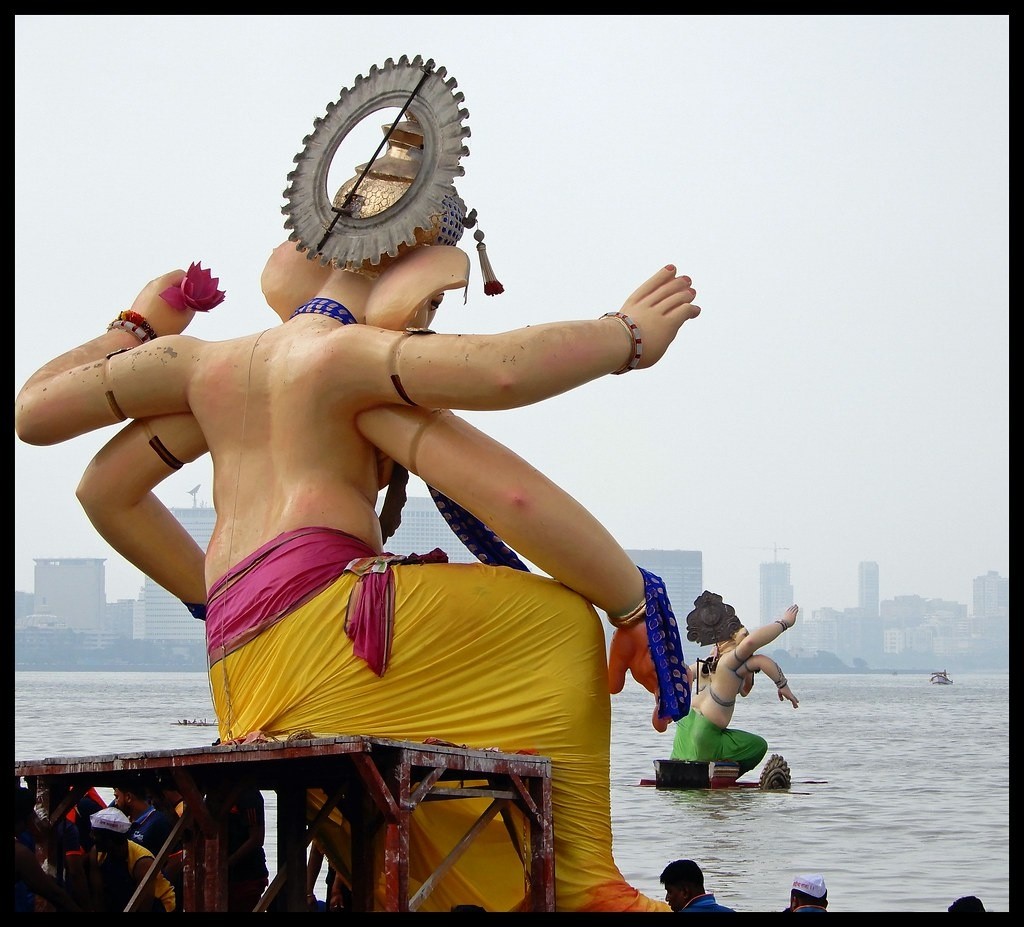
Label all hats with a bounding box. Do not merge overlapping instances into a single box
[793,872,827,898]
[90,807,132,834]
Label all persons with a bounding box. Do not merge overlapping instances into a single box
[14,774,361,912]
[948,895,986,912]
[780,874,829,913]
[659,860,736,911]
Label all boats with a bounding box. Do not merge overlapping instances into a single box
[930,669,954,686]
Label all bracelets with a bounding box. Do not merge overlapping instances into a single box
[776,678,787,690]
[775,618,789,629]
[596,310,644,375]
[606,596,651,629]
[108,310,157,344]
